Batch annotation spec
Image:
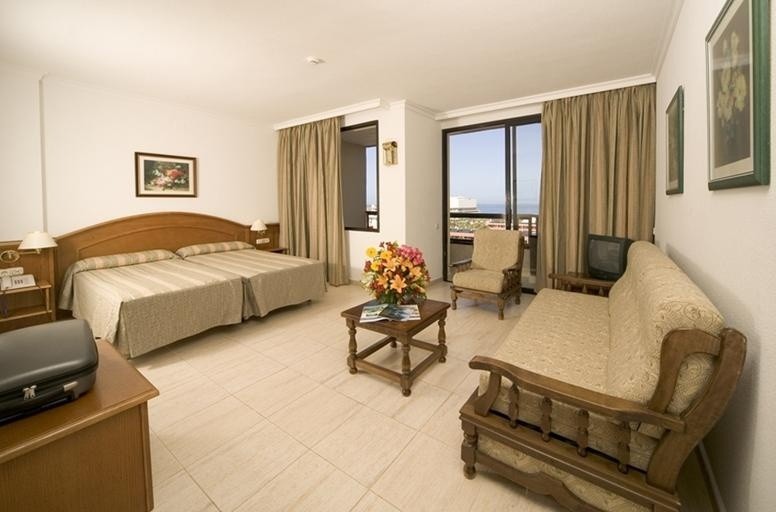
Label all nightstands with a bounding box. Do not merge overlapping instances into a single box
[0,280,55,333]
[268,248,288,253]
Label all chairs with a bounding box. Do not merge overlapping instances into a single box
[449,228,526,320]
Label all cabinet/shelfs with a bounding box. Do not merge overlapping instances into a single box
[0,337,159,512]
[548,271,615,297]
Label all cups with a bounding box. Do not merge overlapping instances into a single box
[414,293,428,306]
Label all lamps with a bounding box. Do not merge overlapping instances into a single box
[0,231,59,264]
[250,221,272,237]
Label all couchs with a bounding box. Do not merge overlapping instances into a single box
[457,239,749,511]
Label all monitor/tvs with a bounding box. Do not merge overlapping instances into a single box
[587,234,635,281]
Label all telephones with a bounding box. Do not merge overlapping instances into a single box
[0,275,36,290]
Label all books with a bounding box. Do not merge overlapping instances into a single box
[359,304,421,323]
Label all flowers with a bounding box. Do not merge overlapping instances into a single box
[361,241,432,305]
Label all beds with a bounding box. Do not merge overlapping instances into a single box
[57,247,244,361]
[175,239,329,322]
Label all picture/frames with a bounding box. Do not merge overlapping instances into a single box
[133,150,197,198]
[704,0,771,192]
[664,85,686,196]
